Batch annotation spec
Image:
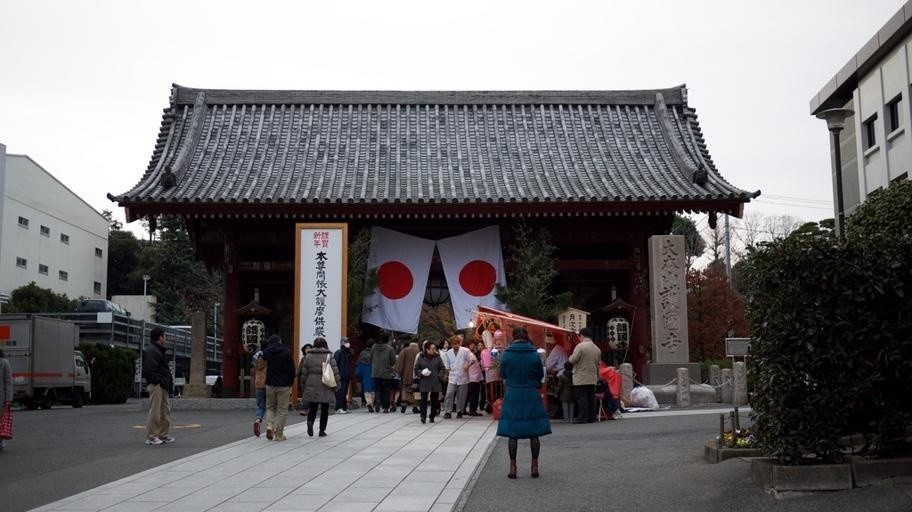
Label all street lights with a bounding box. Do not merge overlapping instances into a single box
[139,275,151,350]
[212,302,221,360]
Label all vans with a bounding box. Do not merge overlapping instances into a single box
[74,298,134,342]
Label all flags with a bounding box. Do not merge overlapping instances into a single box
[435,225,517,331]
[359,224,436,335]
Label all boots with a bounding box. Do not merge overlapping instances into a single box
[508,459,517,479]
[531,458,538,477]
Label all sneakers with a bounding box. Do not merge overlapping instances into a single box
[336,408,352,414]
[307,421,314,437]
[145,437,175,445]
[421,410,483,423]
[254,421,286,441]
[367,402,420,413]
[319,431,326,436]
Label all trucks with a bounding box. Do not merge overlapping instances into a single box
[0,314,96,410]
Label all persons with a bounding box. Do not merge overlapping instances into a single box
[333,337,352,414]
[568,327,601,423]
[300,337,342,437]
[356,331,497,424]
[143,325,179,444]
[296,343,313,415]
[250,335,295,442]
[545,333,568,420]
[498,328,555,478]
[0,348,17,451]
[557,361,574,423]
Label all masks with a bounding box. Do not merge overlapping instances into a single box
[345,343,351,348]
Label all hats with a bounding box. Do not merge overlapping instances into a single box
[545,334,556,344]
[340,337,350,342]
[151,325,166,339]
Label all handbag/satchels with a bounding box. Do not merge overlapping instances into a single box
[321,361,338,388]
